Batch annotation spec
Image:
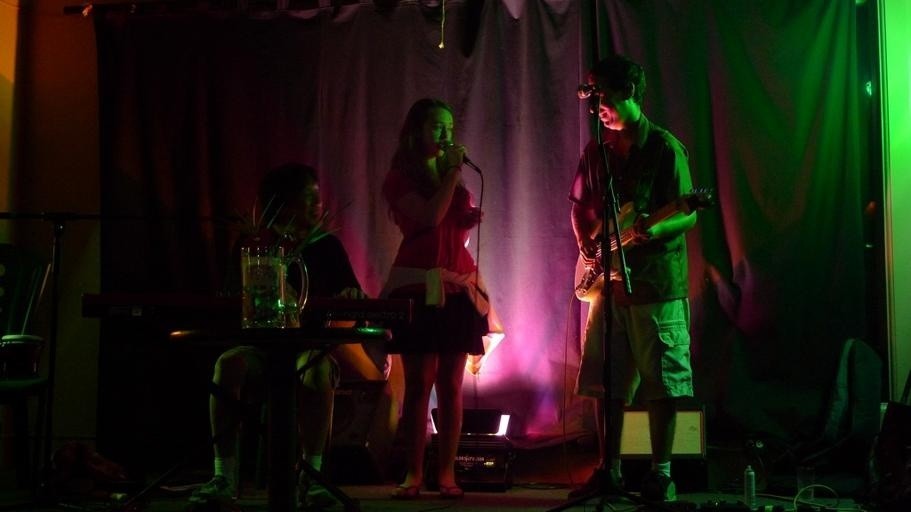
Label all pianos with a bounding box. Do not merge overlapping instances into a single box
[80,290,414,332]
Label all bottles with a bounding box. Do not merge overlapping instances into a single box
[743,465,756,505]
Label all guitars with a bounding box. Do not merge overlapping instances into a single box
[572,185,719,300]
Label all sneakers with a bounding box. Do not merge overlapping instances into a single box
[640,478,677,501]
[299,480,337,508]
[187,475,240,506]
[568,477,625,499]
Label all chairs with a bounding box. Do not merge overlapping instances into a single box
[0,245,48,488]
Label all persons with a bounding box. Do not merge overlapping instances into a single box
[376,95,491,500]
[188,159,369,505]
[569,52,701,502]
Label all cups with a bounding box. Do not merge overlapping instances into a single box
[240,247,309,328]
[796,467,815,502]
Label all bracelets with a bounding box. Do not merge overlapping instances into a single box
[448,163,463,172]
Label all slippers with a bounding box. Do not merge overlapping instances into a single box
[391,484,420,498]
[439,486,464,498]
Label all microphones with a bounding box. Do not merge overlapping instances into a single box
[440,140,482,174]
[577,82,602,99]
